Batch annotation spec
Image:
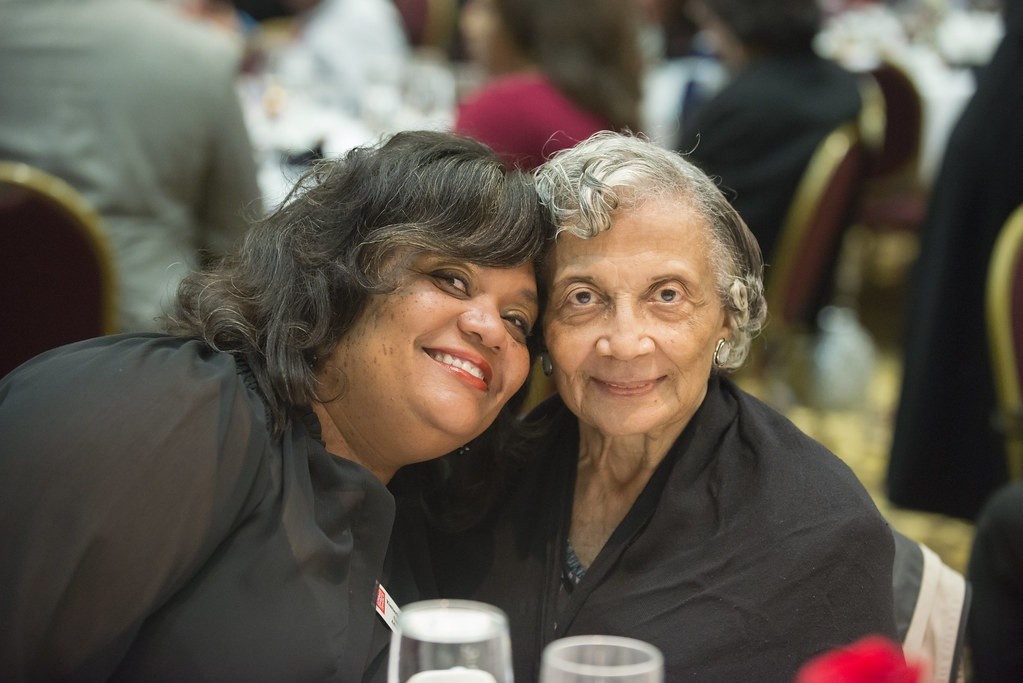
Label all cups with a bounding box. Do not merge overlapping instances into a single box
[388,600,514,683]
[540,636,664,683]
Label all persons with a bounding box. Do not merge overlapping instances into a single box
[673,0,861,305]
[0,0,263,330]
[0,130,549,683]
[385,129,905,683]
[449,0,647,176]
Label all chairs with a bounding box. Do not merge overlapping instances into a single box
[830,39,925,365]
[982,202,1023,482]
[1,158,124,380]
[732,123,873,453]
